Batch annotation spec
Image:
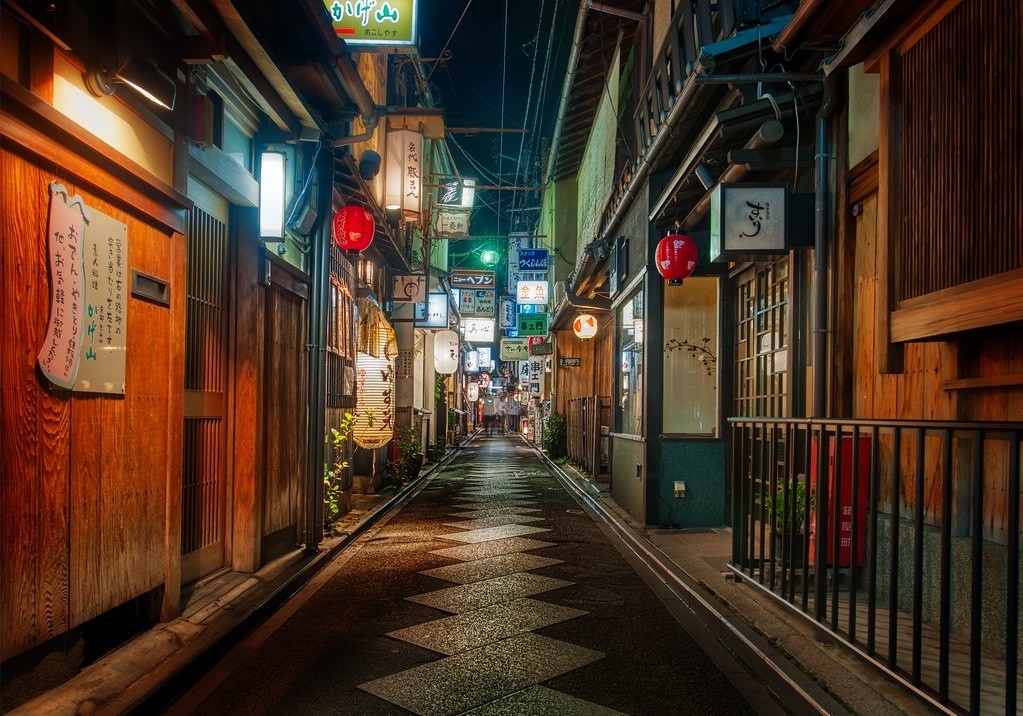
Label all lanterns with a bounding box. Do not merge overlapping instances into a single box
[467,381,479,402]
[477,370,490,388]
[528,355,545,401]
[573,312,599,344]
[350,309,397,453]
[385,116,426,229]
[433,327,460,379]
[332,205,375,257]
[653,228,698,290]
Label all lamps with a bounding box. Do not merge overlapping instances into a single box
[258,150,288,246]
[84,56,176,112]
[384,203,404,231]
[359,150,383,181]
[479,250,500,267]
[355,258,374,289]
[695,163,717,191]
[583,239,614,261]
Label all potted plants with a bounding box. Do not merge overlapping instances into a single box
[753,476,817,570]
[383,423,425,492]
